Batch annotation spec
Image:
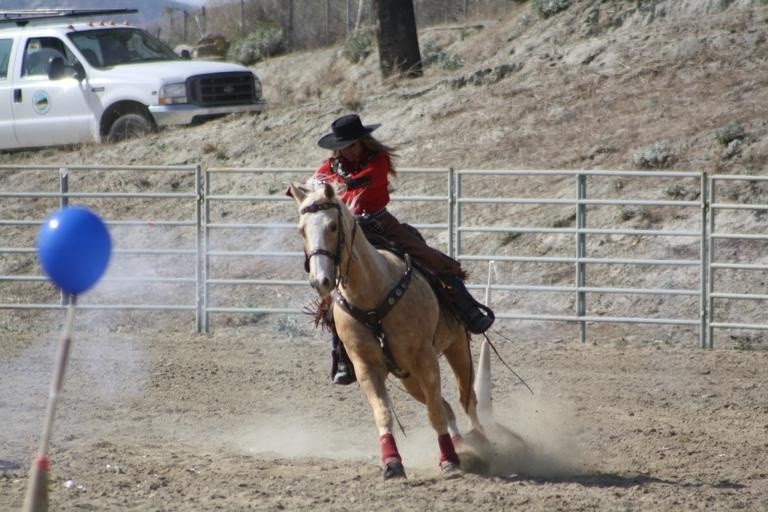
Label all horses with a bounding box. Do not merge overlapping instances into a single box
[288,181,490,480]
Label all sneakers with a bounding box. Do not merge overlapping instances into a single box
[466,306,492,330]
[334,363,351,384]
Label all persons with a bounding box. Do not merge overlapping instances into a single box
[180,49,192,61]
[302,112,493,384]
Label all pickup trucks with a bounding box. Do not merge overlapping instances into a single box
[0,19,266,155]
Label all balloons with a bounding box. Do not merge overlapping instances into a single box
[33,205,114,298]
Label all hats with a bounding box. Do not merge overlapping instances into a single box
[319,115,381,150]
